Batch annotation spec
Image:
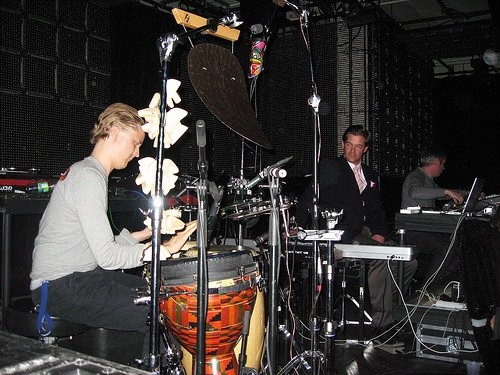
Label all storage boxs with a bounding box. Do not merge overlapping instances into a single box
[416,323,481,363]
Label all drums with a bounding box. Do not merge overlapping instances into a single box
[218,194,291,220]
[143,240,266,375]
[144,249,257,375]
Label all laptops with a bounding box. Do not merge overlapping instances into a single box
[433,177,487,215]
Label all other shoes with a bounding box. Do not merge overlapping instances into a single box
[470,305,496,320]
[373,327,400,345]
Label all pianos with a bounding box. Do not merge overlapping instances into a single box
[212,237,419,349]
[0,166,200,332]
[395,194,499,311]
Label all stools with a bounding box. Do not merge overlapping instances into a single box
[5,298,90,347]
[333,258,373,328]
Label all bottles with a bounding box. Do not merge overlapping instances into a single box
[26,179,58,194]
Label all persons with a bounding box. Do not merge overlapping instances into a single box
[298,125,417,341]
[31,103,198,361]
[402,148,484,310]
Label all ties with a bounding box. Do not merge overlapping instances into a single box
[353,166,365,194]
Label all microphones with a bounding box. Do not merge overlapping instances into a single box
[243,165,277,189]
[254,219,293,245]
